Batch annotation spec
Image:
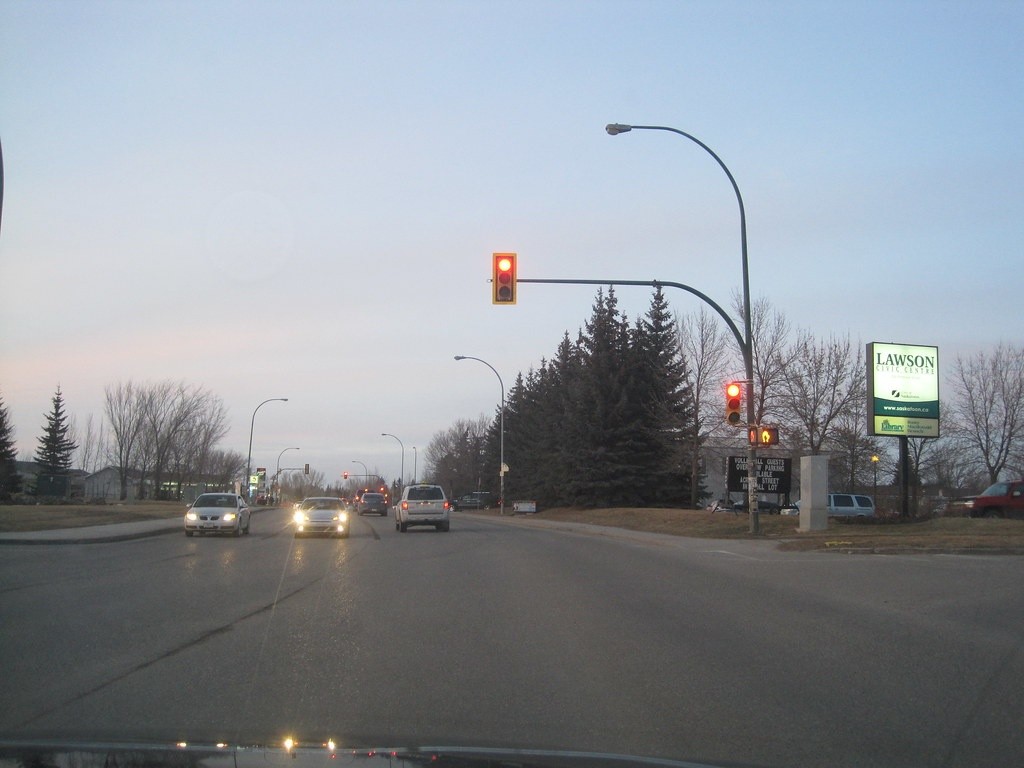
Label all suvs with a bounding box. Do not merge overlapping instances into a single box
[352,488,377,511]
[962,479,1024,518]
[357,493,388,516]
[392,482,450,532]
[455,491,502,510]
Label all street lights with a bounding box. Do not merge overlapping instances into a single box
[352,461,368,485]
[245,398,290,499]
[452,353,506,514]
[276,447,301,496]
[412,446,417,482]
[871,455,878,506]
[382,433,404,485]
[604,121,762,532]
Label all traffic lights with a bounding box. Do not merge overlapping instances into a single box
[344,472,348,479]
[726,383,744,426]
[492,252,518,303]
[750,428,780,444]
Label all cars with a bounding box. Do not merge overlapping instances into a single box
[184,492,251,537]
[734,500,781,515]
[292,496,350,538]
[293,501,303,510]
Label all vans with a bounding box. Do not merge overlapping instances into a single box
[793,492,877,517]
